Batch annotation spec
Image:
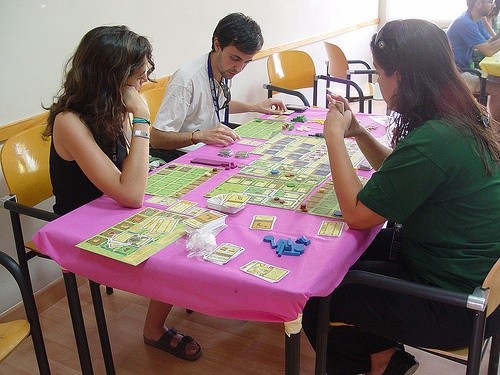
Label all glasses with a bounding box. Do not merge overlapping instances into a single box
[217,82,231,110]
[374,21,393,55]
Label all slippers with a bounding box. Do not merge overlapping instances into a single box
[143,327,202,361]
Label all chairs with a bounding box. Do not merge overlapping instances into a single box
[0,116,193,375]
[314,252,500,375]
[323,41,391,117]
[0,252,52,375]
[457,67,486,110]
[261,50,364,115]
[139,72,242,164]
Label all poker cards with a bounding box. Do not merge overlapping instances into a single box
[249,214,277,231]
[108,192,251,249]
[317,220,344,237]
[334,100,344,116]
[203,241,245,265]
[239,259,291,284]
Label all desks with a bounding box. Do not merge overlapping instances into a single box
[31,105,410,375]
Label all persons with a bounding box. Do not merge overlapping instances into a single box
[445,0,500,122]
[37,26,203,362]
[148,12,287,153]
[302,19,500,375]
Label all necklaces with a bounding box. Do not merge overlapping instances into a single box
[218,75,223,86]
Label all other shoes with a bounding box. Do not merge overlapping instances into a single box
[382,350,420,375]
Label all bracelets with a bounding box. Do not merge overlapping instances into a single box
[188,128,200,145]
[130,116,150,125]
[130,129,151,140]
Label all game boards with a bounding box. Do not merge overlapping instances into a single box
[233,117,288,141]
[76,207,194,268]
[144,162,224,199]
[205,132,390,221]
